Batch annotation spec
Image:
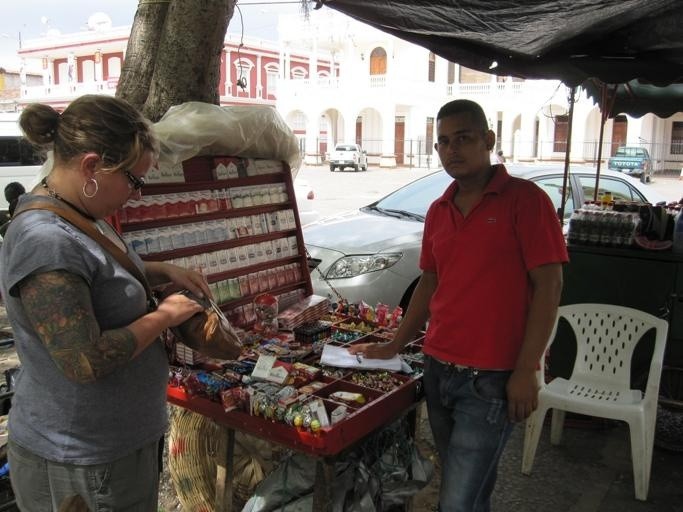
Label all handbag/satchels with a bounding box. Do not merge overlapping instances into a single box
[154,281,243,363]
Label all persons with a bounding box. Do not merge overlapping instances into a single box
[496,149,506,164]
[1,92,213,510]
[352,99,568,512]
[1,180,25,239]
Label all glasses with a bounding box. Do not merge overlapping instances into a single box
[101,157,146,192]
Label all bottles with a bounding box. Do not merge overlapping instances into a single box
[566,192,683,247]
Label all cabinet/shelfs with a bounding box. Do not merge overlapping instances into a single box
[110,156,313,332]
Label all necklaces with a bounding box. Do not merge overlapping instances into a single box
[40,175,98,223]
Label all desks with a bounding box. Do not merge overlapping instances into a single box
[550,235,683,408]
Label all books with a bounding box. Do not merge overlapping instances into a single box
[318,342,403,373]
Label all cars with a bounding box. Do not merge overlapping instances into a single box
[0,114,51,211]
[302,162,663,353]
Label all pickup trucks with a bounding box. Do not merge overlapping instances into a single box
[329,143,367,171]
[607,146,654,184]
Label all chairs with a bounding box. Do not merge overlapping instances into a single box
[519,302,671,502]
